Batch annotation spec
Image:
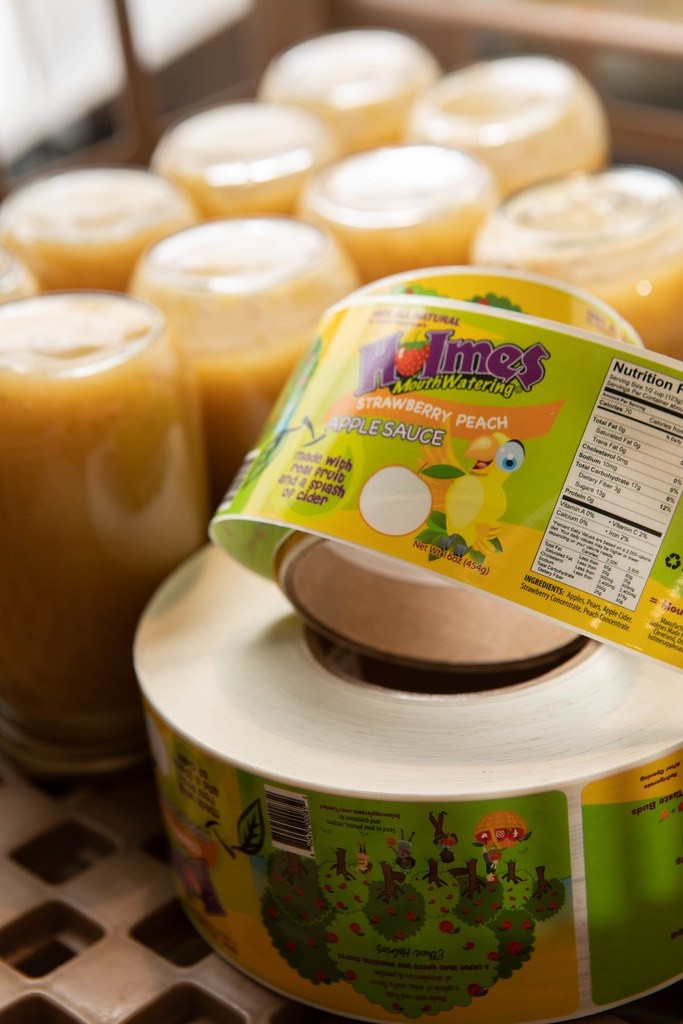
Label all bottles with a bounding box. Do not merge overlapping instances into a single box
[0,27,680,797]
